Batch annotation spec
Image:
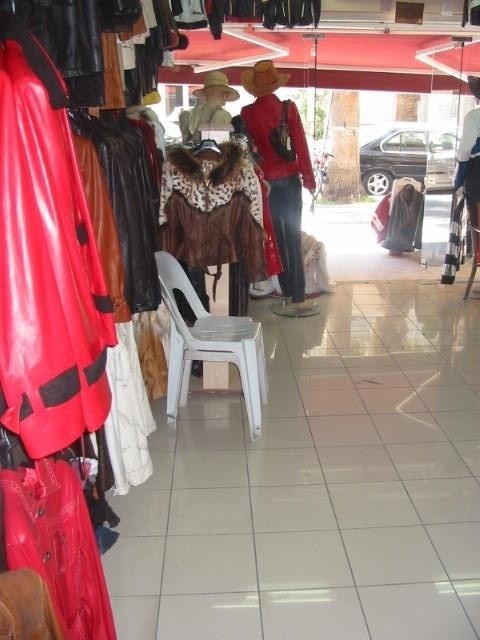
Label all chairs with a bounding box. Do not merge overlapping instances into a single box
[461,179,480,301]
[154,249,270,442]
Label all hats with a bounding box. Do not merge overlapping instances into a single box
[193,71,239,101]
[241,60,291,97]
[468,76,479,99]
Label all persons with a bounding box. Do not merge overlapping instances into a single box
[452,75,480,266]
[179,70,240,143]
[240,59,317,303]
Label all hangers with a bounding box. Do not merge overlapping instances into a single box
[67,95,133,147]
[188,119,224,158]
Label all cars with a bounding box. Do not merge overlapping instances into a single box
[360,129,475,197]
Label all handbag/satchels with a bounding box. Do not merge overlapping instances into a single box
[270,123,296,161]
